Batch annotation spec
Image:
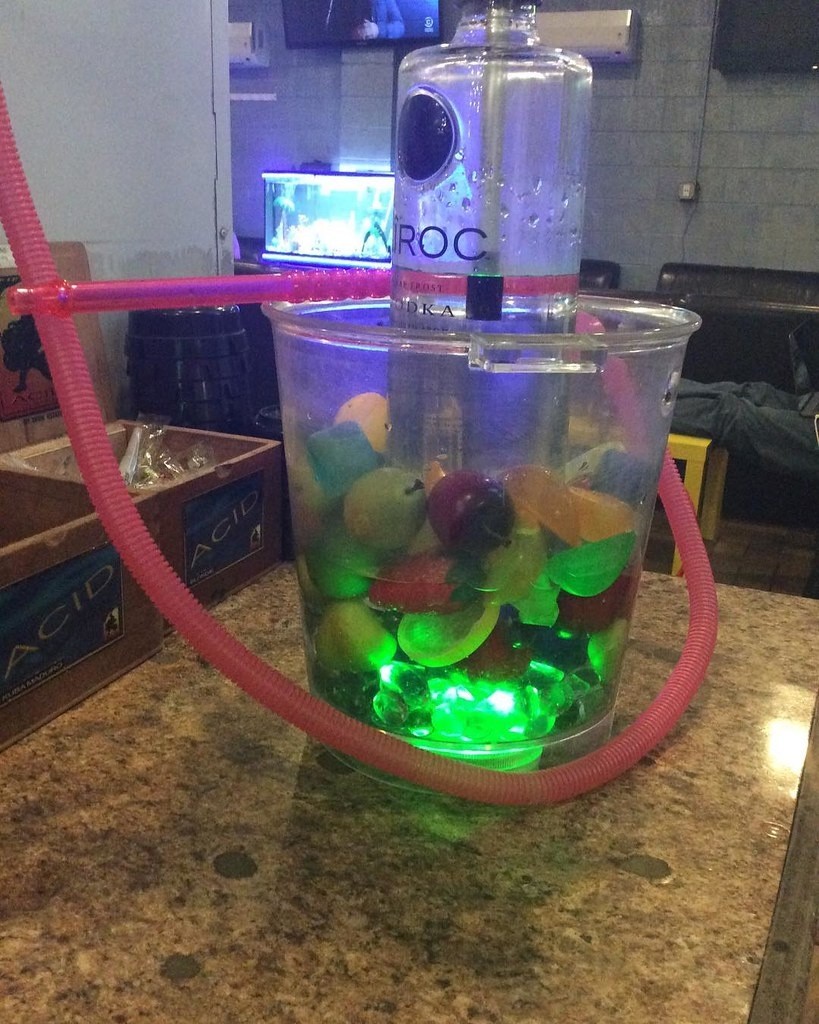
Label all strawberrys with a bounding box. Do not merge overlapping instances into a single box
[365,554,483,612]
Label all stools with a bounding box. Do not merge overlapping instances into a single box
[664,434,728,577]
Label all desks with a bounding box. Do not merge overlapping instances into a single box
[0,566,819,1024]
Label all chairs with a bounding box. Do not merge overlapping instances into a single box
[578,259,620,291]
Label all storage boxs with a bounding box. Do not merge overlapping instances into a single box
[0,419,282,754]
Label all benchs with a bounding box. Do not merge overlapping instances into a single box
[656,264,819,518]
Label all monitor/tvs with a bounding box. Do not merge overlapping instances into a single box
[713,0,819,74]
[281,0,445,50]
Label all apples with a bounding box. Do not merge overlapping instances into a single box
[285,393,636,673]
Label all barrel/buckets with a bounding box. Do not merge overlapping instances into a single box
[230,405,292,545]
[116,305,254,437]
